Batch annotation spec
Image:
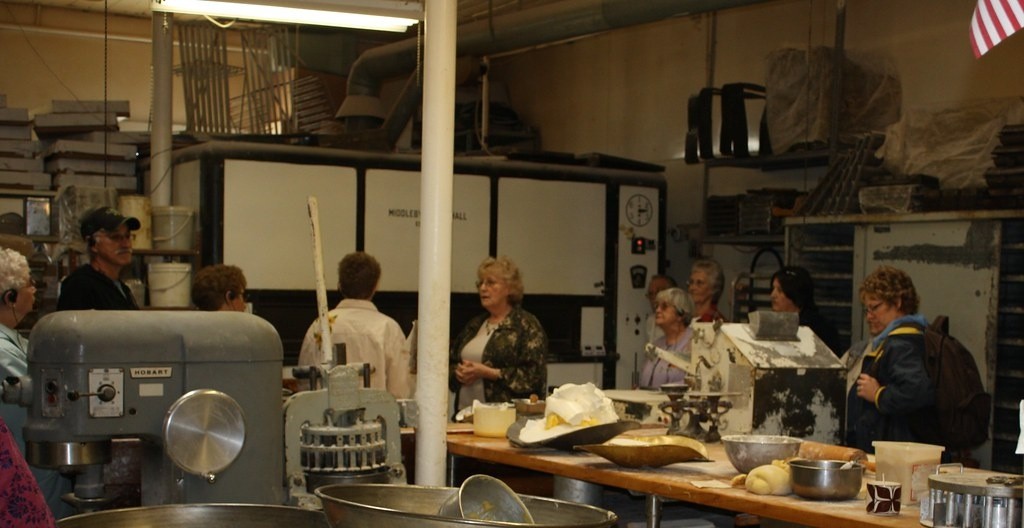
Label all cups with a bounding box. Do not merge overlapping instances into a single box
[866,481,901,515]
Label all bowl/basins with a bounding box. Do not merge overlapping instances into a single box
[507,418,642,452]
[721,434,802,474]
[511,398,546,414]
[788,459,863,499]
[575,435,713,467]
[437,475,535,524]
[471,405,518,438]
[53,503,328,528]
[624,424,669,434]
[314,483,619,528]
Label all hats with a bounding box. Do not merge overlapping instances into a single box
[80,206,141,242]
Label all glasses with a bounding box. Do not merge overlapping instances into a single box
[15,276,37,290]
[686,277,707,288]
[475,275,505,289]
[222,287,251,303]
[96,229,136,245]
[652,299,675,311]
[862,300,889,317]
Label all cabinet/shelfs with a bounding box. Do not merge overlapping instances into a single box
[700,147,838,243]
[782,208,1024,477]
[0,187,200,311]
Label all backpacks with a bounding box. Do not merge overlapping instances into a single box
[892,315,991,450]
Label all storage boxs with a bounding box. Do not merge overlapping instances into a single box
[857,182,942,212]
[0,93,150,194]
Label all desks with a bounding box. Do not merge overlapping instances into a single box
[400,422,473,454]
[443,434,938,528]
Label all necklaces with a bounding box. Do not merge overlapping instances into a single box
[485,318,496,335]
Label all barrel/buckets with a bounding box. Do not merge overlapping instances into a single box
[151,206,195,249]
[147,263,193,307]
[119,195,153,248]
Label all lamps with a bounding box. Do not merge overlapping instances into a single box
[152,0,425,33]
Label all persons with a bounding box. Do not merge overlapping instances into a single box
[640,288,695,389]
[685,256,729,323]
[838,265,960,465]
[0,247,73,521]
[190,265,248,312]
[769,265,841,356]
[643,274,679,341]
[450,257,547,488]
[56,206,140,311]
[296,252,411,402]
